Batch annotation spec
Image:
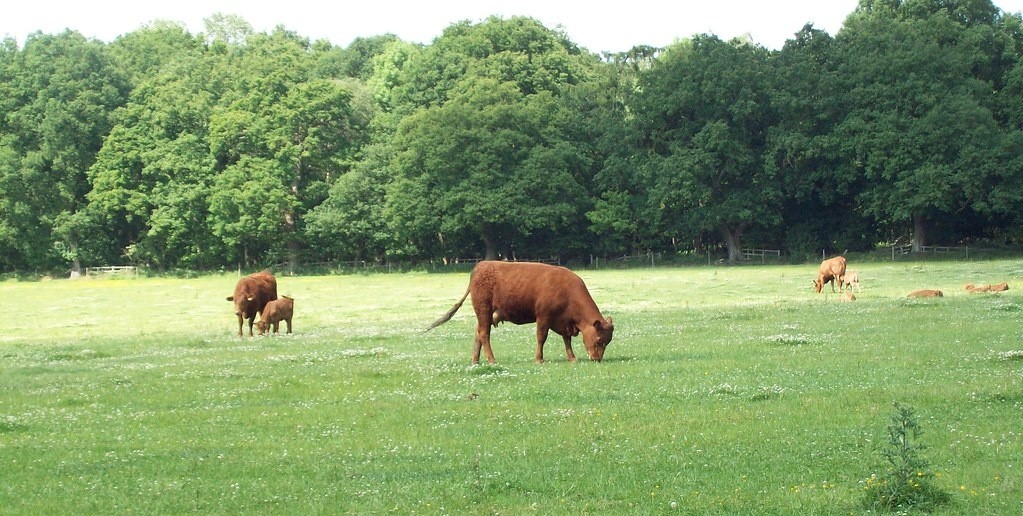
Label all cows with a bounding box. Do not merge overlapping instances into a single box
[812,255,862,295]
[226,270,277,337]
[250,295,294,335]
[424,260,614,364]
[964,284,1008,291]
[906,290,943,299]
